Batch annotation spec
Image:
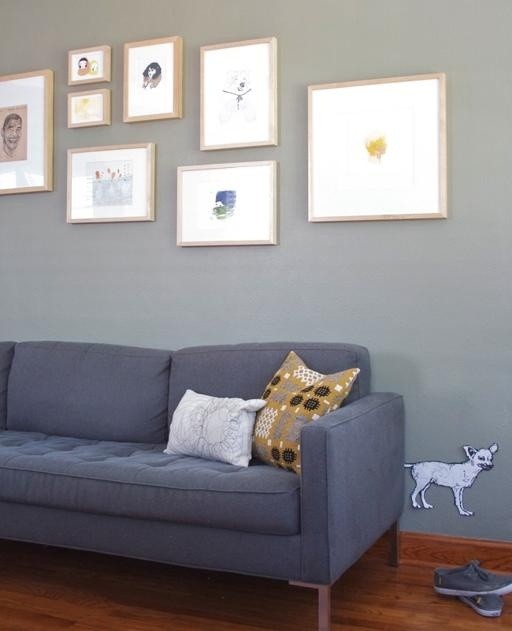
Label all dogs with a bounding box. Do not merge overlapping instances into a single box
[403,440,500,516]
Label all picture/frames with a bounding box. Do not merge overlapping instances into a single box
[177,160,278,246]
[199,36,278,151]
[66,46,111,84]
[67,88,112,129]
[122,34,182,123]
[308,73,448,223]
[1,68,53,194]
[65,141,156,224]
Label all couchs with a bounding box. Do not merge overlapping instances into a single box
[0,391,406,631]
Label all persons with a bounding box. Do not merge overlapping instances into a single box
[0,113,24,161]
[142,62,164,89]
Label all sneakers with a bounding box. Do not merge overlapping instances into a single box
[434,559,512,616]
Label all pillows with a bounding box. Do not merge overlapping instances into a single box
[163,389,267,468]
[0,341,18,429]
[7,341,173,441]
[166,342,372,443]
[250,351,360,476]
